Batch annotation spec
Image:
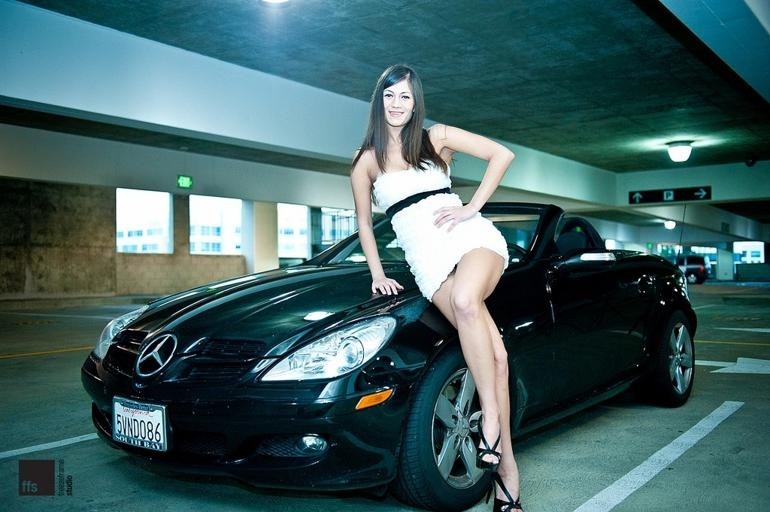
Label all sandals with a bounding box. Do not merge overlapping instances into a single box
[476,414,502,472]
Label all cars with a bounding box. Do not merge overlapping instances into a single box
[671,252,711,286]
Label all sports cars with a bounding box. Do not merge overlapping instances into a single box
[80,201,698,512]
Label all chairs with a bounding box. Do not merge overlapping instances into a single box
[556,230,589,254]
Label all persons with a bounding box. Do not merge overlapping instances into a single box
[346,62,527,512]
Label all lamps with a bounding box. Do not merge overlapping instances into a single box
[665,142,693,163]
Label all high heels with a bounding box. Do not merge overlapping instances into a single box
[486,470,524,512]
